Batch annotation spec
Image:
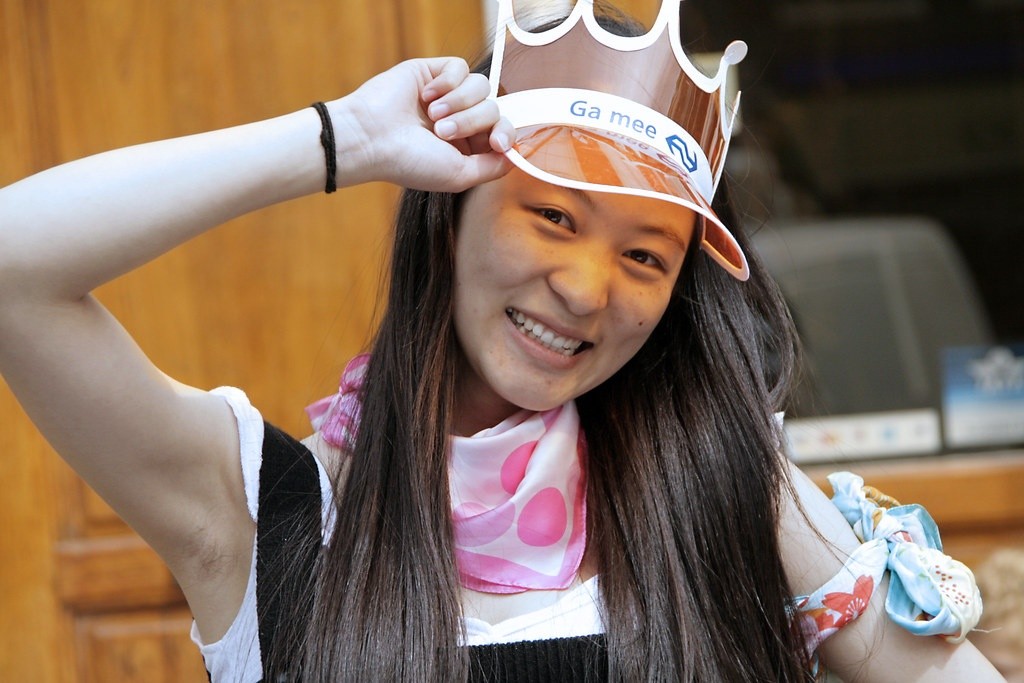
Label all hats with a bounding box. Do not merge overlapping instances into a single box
[487,0,750,282]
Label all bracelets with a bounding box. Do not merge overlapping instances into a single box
[310,101,337,194]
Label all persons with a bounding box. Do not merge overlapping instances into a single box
[0,0,1007,683]
